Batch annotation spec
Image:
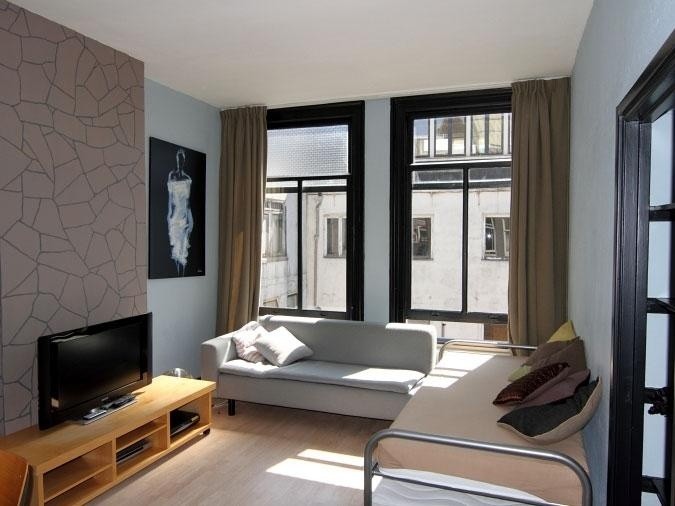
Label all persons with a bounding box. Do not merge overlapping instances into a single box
[165,148,194,277]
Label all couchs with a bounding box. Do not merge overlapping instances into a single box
[200,315,437,421]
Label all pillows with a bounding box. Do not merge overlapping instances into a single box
[493,320,603,445]
[231,321,270,362]
[254,326,314,366]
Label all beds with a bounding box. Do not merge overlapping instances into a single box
[362,339,593,506]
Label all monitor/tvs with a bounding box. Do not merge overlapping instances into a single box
[38,312,153,430]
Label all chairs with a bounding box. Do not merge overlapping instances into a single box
[0,447,28,506]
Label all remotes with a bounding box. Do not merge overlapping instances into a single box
[112,396,134,407]
[83,410,107,419]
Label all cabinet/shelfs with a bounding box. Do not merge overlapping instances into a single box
[0,374,217,506]
